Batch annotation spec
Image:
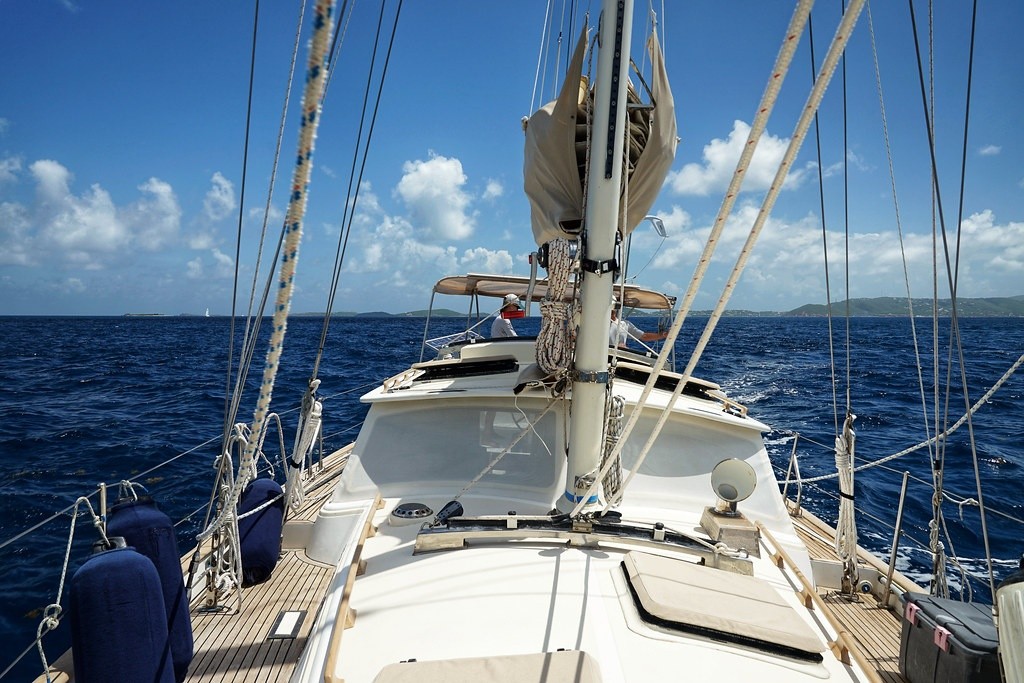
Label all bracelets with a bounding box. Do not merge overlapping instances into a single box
[663,332,667,337]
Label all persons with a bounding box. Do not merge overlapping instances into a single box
[609,295,671,349]
[491,294,525,338]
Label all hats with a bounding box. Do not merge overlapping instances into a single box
[503,294,525,310]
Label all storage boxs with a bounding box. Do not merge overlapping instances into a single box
[898,591,1003,683]
